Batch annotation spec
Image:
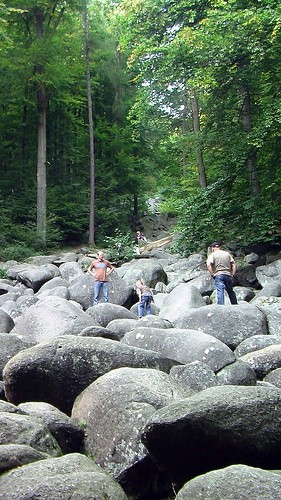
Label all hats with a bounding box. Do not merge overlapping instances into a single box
[209,242,219,247]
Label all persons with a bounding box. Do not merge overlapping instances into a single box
[137,230,147,243]
[135,280,155,318]
[207,241,239,305]
[88,252,114,304]
